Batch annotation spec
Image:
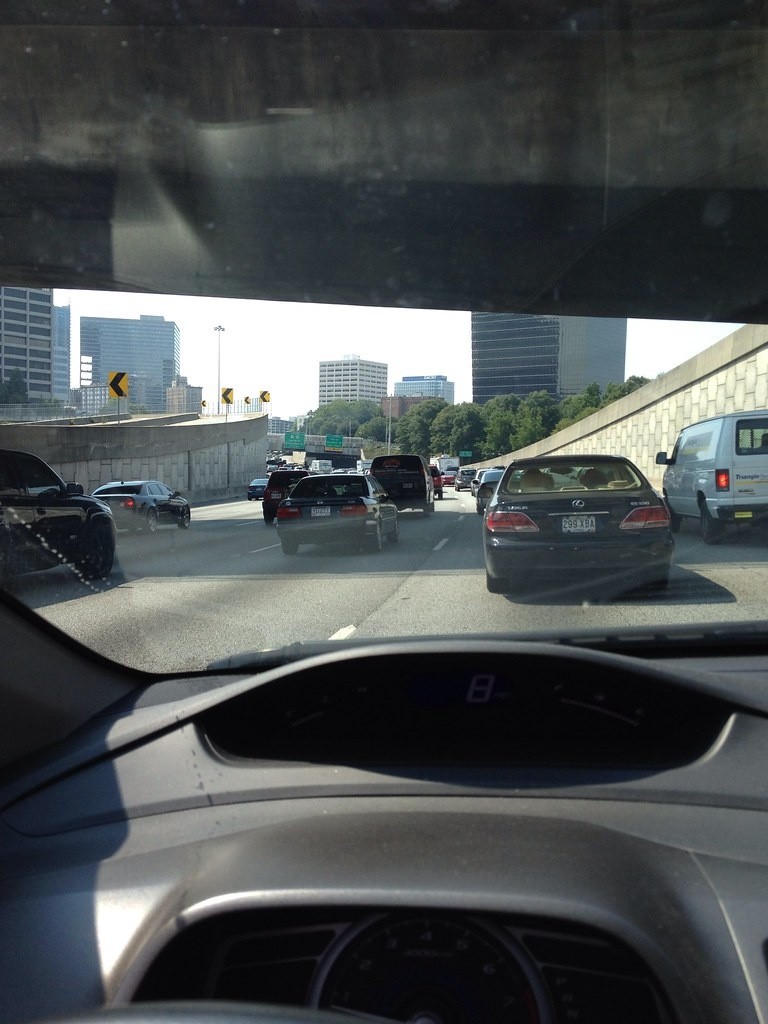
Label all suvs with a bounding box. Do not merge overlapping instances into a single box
[262,470,310,526]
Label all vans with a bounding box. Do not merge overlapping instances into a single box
[370,454,434,517]
[655,408,768,545]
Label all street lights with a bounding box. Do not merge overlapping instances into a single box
[213,324,225,415]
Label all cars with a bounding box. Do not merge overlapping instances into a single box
[272,474,400,554]
[428,463,506,515]
[0,448,117,582]
[90,479,191,535]
[481,454,675,594]
[247,460,357,503]
